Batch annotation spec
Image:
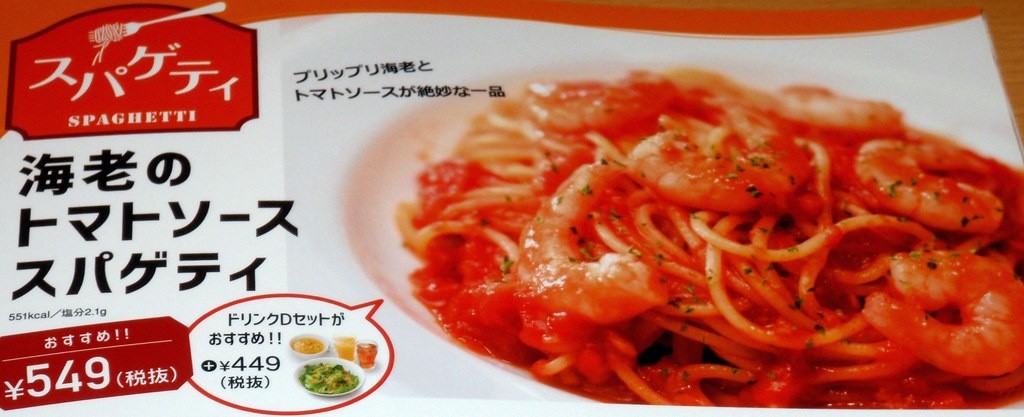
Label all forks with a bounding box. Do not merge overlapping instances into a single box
[89,2,226,41]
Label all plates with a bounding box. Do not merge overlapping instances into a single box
[336,61,1024,407]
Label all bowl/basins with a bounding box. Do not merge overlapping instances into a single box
[292,356,365,396]
[288,332,329,359]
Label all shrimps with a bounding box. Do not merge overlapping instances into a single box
[420,66,1023,408]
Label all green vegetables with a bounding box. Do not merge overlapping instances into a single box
[303,365,357,395]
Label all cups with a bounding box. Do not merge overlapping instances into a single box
[335,335,355,362]
[355,338,378,370]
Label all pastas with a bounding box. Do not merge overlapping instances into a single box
[398,70,1024,409]
